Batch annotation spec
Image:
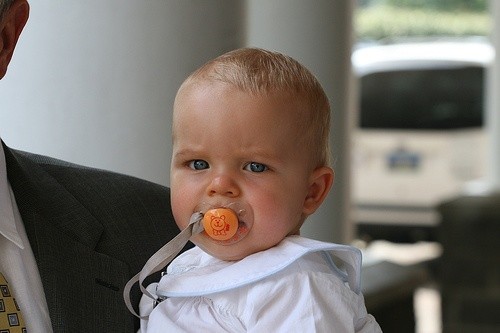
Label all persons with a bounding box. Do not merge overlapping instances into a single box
[136,47,383,333]
[0,0,196,333]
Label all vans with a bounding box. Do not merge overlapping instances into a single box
[349,38,499,245]
